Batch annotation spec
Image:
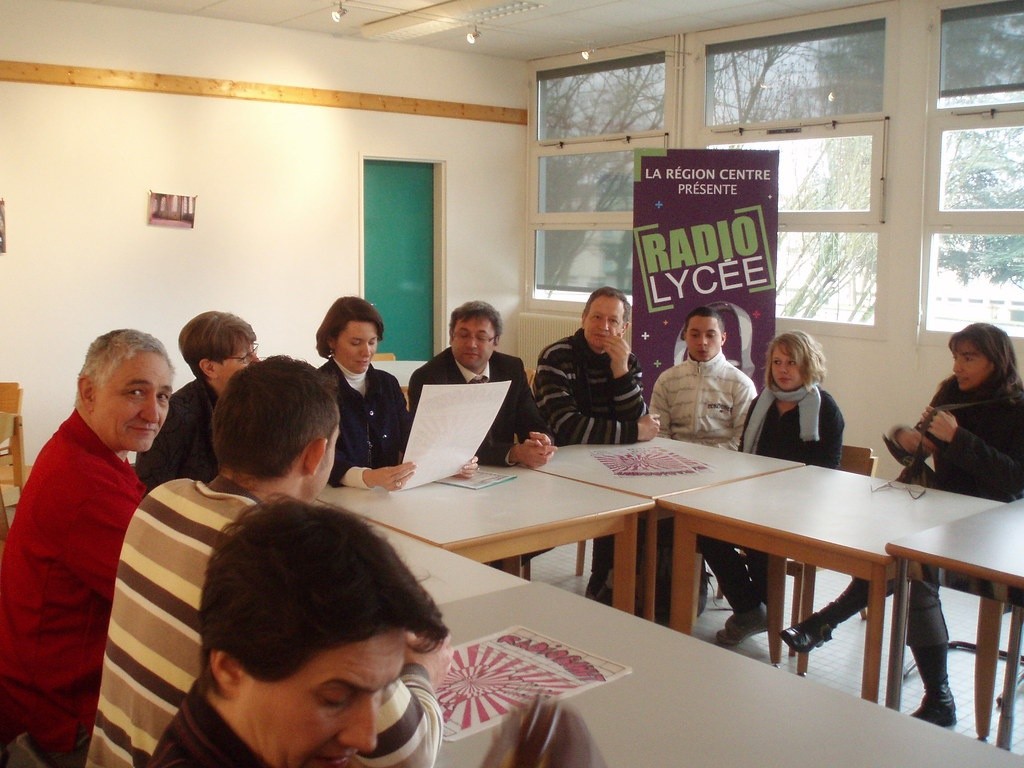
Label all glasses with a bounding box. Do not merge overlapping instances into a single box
[229,343,259,363]
[452,332,497,345]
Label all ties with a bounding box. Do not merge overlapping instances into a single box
[471,376,487,384]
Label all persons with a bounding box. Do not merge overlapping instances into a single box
[408,287,661,620]
[649,307,845,644]
[780,323,1024,726]
[312,296,478,491]
[0,311,447,768]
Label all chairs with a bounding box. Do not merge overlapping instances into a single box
[784,445,878,656]
[0,381,26,496]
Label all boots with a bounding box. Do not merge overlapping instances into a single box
[778,602,844,651]
[910,683,956,726]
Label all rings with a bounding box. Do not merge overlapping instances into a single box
[395,479,402,489]
[476,467,480,471]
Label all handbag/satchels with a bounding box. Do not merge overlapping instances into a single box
[896,460,936,489]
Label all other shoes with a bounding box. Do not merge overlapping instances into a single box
[715,614,766,642]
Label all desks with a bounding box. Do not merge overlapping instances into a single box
[886,497,1024,752]
[657,465,1012,739]
[319,462,655,613]
[518,434,805,623]
[370,524,1024,768]
